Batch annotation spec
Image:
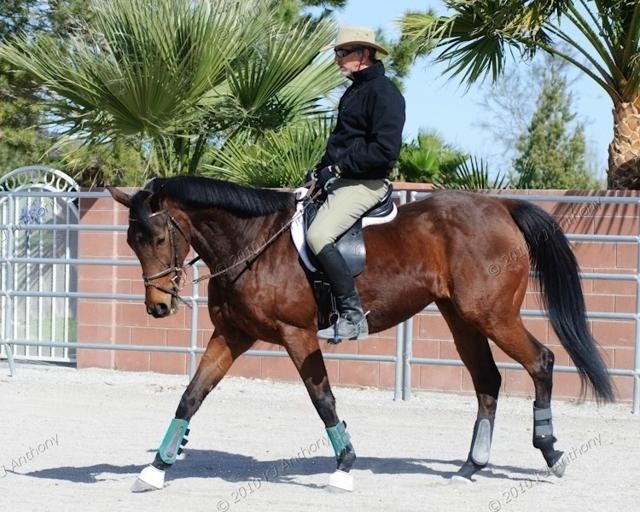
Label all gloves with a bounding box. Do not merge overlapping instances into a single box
[319,165,340,193]
[307,162,327,184]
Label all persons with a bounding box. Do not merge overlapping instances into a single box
[305,25,405,341]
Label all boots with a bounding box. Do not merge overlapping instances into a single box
[314,246,369,341]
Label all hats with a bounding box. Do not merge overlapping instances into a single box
[319,26,389,55]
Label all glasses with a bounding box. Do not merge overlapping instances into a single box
[333,48,357,57]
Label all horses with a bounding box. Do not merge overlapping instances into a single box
[106,175,622,493]
